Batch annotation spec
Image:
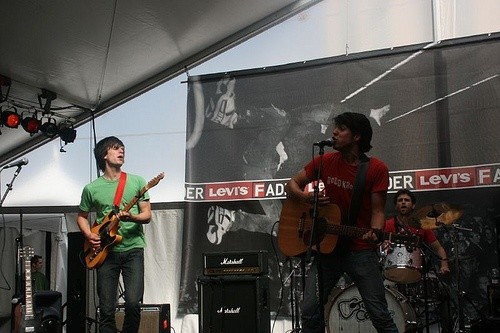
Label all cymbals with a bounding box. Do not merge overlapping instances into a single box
[408,201,463,230]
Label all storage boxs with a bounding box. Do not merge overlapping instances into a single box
[197,274,270,333]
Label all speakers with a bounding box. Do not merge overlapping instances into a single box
[197,275,271,333]
[115,304,171,333]
[65,232,99,333]
[32,291,63,333]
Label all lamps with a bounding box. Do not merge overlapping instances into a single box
[0,104,76,147]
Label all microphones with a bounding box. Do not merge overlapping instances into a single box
[313,138,334,147]
[3,157,28,168]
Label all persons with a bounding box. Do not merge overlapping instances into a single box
[204,77,391,246]
[77,136,152,333]
[285,111,449,333]
[18,255,48,292]
[434,216,500,312]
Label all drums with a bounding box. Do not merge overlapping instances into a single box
[378,236,425,282]
[328,281,416,333]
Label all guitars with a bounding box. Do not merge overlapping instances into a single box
[276,194,422,258]
[83,174,163,271]
[14,246,45,333]
